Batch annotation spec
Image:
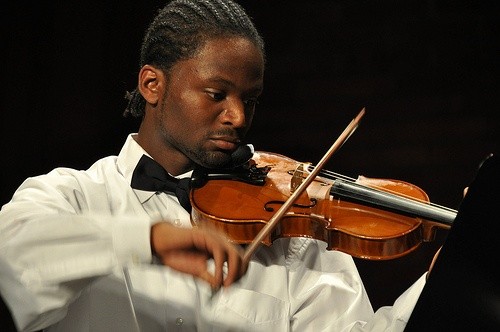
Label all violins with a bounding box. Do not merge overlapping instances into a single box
[187,148,459,262]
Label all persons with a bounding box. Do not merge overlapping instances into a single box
[0,2,471,332]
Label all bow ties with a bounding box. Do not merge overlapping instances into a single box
[500,289,500,292]
[129,155,195,213]
